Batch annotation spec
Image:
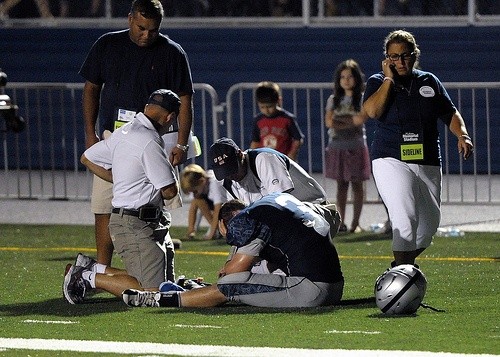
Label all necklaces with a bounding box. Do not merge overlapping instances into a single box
[400,73,416,96]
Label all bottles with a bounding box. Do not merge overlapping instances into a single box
[434,228,465,237]
[365,223,385,233]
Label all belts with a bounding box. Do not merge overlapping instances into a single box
[112,208,140,217]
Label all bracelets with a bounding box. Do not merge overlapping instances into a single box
[458,135,471,140]
[384,77,395,85]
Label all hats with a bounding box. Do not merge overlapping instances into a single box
[147,88,181,116]
[208,137,240,181]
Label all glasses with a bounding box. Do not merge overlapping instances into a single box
[387,52,415,60]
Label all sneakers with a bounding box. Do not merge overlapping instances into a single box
[75,252,97,271]
[178,277,204,289]
[159,282,189,293]
[62,264,88,305]
[121,288,162,308]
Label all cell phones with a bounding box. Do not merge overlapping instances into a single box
[138,207,160,220]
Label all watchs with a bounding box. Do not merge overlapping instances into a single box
[176,143,190,153]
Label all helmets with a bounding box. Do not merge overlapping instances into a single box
[374,263,428,315]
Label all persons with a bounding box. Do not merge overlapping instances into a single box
[208,137,341,243]
[180,163,263,241]
[362,29,474,268]
[77,0,195,268]
[321,59,370,234]
[121,191,344,311]
[248,83,304,163]
[62,88,181,305]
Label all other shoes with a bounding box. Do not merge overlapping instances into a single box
[339,223,359,233]
